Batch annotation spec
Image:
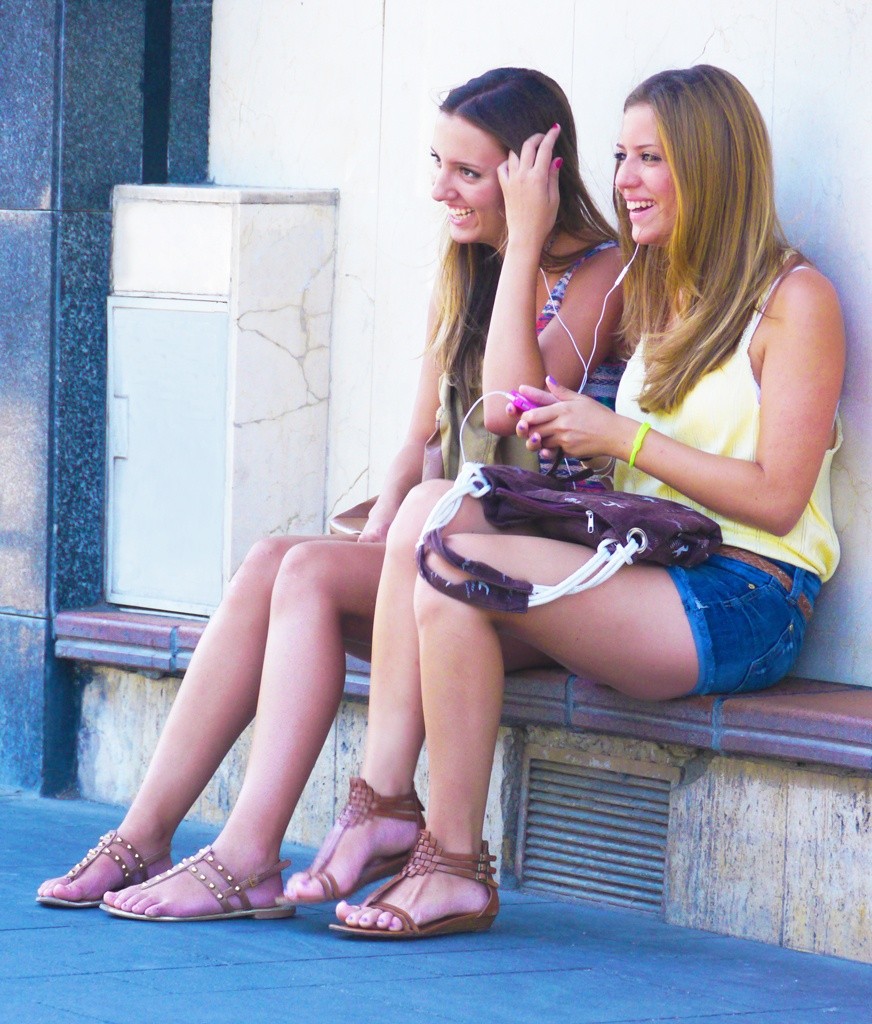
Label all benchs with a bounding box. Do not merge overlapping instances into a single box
[53,614,872,968]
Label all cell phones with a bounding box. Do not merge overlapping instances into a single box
[509,389,538,412]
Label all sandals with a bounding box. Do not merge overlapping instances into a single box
[328,827,499,939]
[99,843,297,921]
[274,777,426,906]
[35,829,171,908]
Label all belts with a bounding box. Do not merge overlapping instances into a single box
[713,543,813,623]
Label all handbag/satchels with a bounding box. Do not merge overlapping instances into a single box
[414,457,723,613]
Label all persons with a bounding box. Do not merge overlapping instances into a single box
[35,63,632,922]
[277,65,846,938]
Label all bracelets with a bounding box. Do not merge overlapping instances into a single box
[580,457,612,473]
[630,422,650,468]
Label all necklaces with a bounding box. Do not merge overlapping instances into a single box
[547,226,560,253]
